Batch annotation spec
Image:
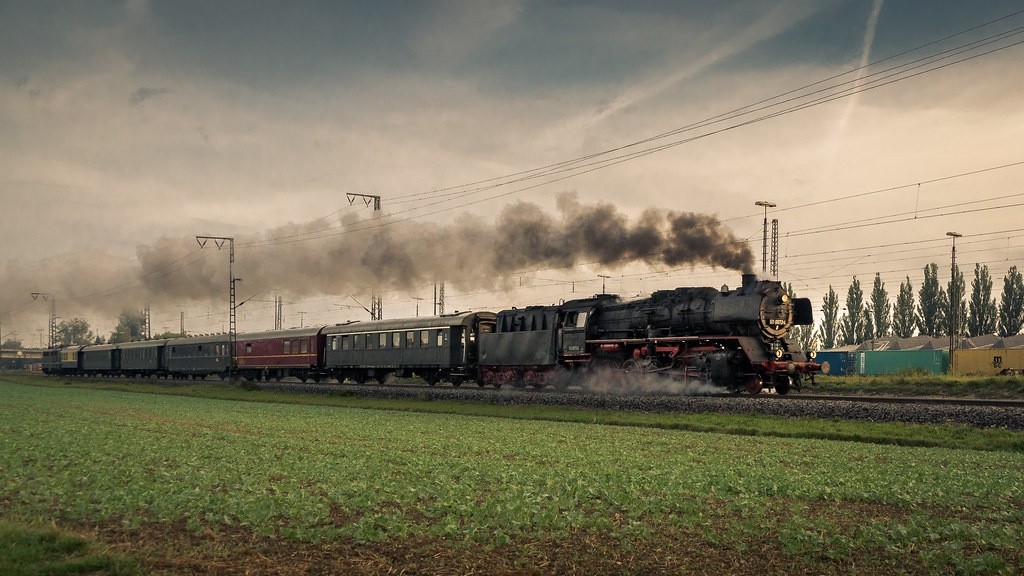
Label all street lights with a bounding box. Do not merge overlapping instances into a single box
[754,201,777,278]
[944,231,964,372]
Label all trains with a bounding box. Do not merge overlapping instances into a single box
[40,272,831,397]
